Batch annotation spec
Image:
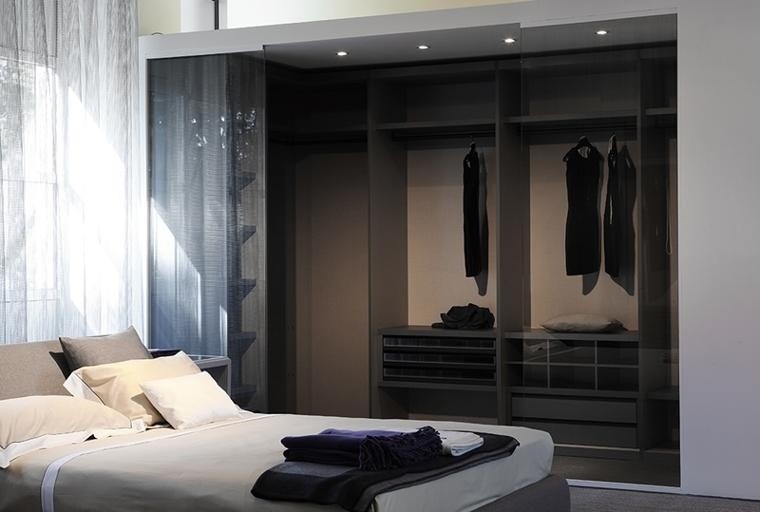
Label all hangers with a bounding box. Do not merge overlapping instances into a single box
[562,129,630,162]
[463,136,479,162]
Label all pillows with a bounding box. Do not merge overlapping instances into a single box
[141,371,242,431]
[63,350,201,429]
[0,394,146,468]
[58,325,152,371]
[539,313,624,332]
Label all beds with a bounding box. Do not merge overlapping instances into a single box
[0,334,570,512]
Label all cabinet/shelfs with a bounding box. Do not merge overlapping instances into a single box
[266,40,680,453]
